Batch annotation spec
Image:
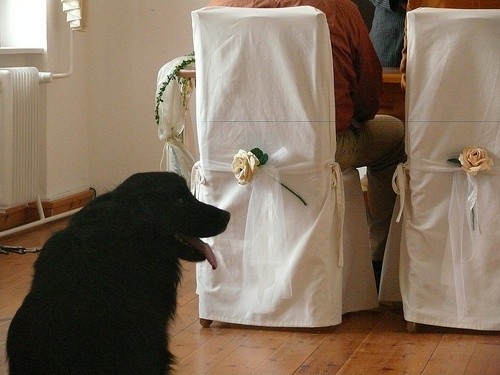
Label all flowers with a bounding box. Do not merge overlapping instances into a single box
[447,146,495,234]
[231,146,309,207]
[153,54,196,125]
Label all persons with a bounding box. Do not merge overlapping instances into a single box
[359,0,409,69]
[216,0,407,277]
[398,0,500,104]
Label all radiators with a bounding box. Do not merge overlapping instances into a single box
[0,63,51,217]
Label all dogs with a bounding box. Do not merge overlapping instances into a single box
[5,172,229,375]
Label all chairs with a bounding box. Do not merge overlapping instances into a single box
[186,4,378,333]
[373,6,500,335]
